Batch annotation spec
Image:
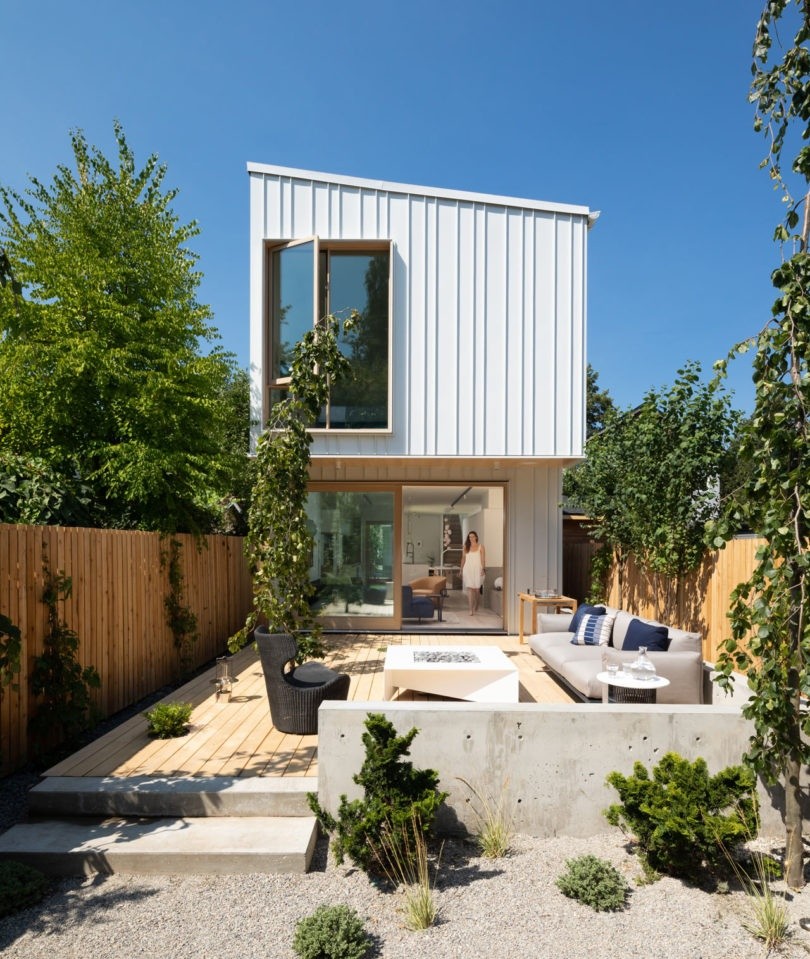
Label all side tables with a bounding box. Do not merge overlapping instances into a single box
[596,670,670,704]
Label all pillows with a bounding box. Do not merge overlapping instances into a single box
[569,613,614,647]
[622,619,668,651]
[568,603,606,633]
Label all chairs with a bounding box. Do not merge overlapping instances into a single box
[408,576,447,610]
[255,624,351,736]
[401,585,434,624]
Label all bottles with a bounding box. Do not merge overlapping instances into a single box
[630,646,657,681]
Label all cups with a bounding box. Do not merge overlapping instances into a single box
[622,661,632,674]
[606,663,619,680]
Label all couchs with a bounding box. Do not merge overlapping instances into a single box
[529,603,704,705]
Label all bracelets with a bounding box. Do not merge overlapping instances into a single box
[482,569,485,571]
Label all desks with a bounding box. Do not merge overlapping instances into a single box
[413,593,442,622]
[517,592,577,644]
[383,645,520,702]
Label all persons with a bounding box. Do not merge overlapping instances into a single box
[457,531,486,617]
